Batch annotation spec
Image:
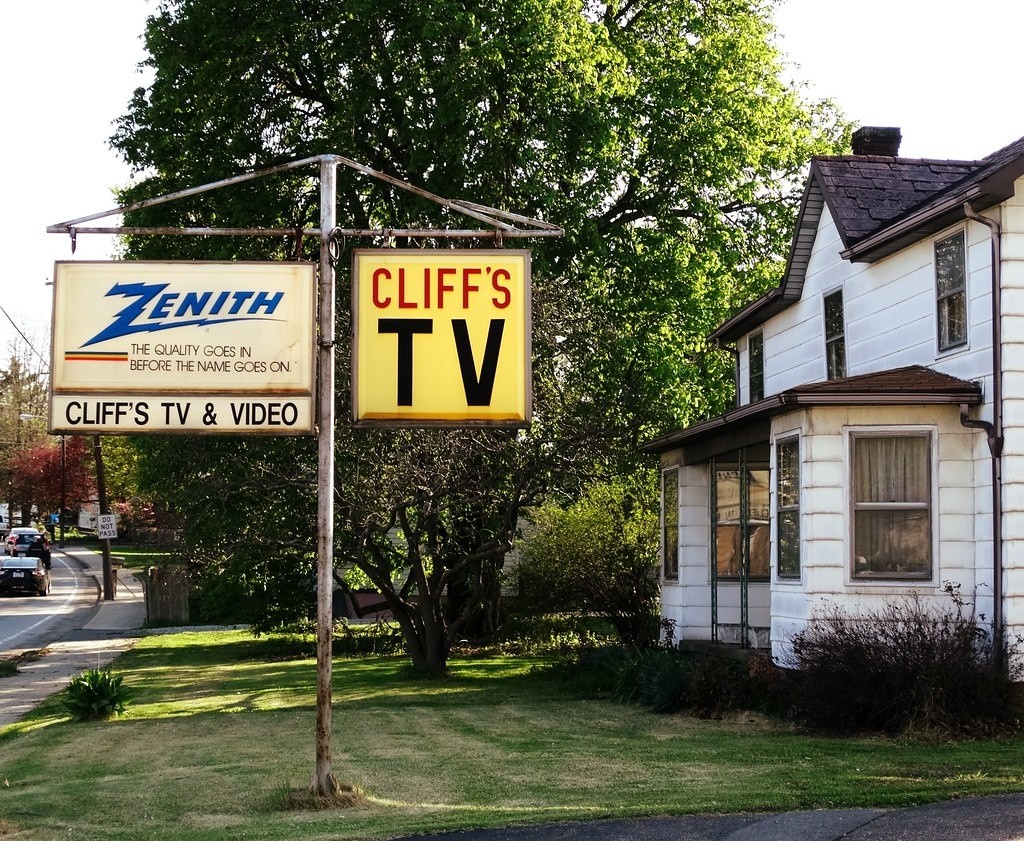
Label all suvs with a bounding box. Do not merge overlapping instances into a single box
[8,533,52,570]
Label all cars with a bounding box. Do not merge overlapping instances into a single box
[0,522,10,542]
[0,556,52,597]
[4,528,39,555]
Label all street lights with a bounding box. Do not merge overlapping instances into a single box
[19,413,67,549]
